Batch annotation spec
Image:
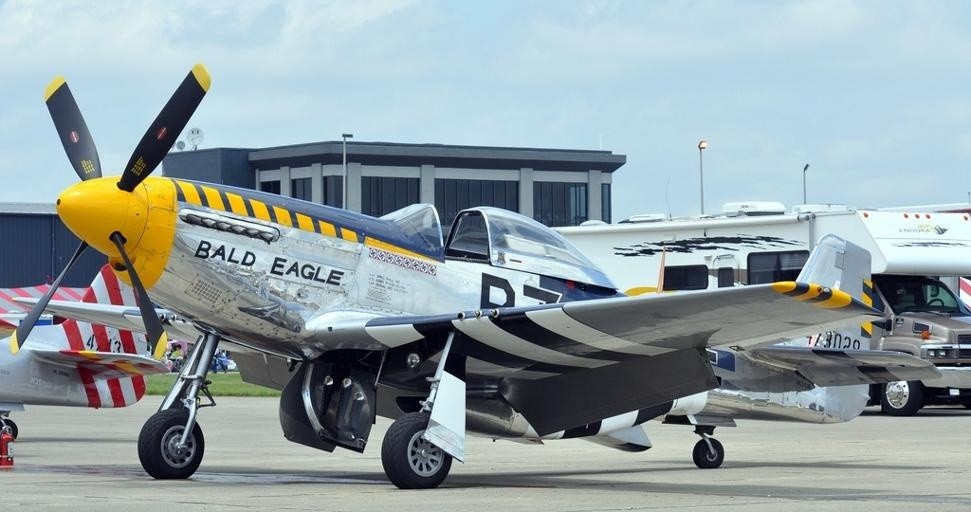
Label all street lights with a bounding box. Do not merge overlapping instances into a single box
[339,132,354,210]
[801,161,812,204]
[697,141,708,213]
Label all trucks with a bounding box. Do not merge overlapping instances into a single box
[542,204,970,416]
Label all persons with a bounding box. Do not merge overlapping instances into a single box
[146,339,227,374]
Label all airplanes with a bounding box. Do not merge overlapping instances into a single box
[11,63,941,491]
[1,263,172,441]
